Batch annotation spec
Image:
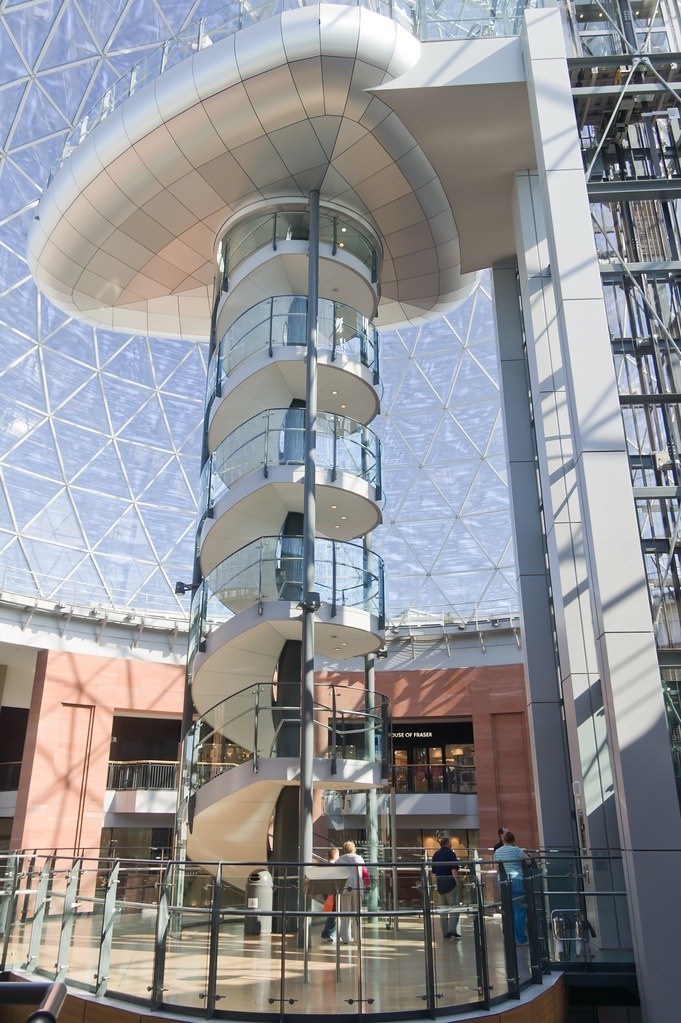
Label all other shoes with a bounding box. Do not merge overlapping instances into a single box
[321,933,333,941]
[444,932,460,938]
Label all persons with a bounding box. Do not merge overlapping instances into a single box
[492,831,531,945]
[431,838,463,938]
[494,826,515,856]
[321,848,340,941]
[335,840,367,944]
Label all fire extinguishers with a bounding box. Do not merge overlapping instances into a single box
[574,910,597,962]
[552,913,572,962]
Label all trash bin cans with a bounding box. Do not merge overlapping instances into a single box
[244,868,273,935]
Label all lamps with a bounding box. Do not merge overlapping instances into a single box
[304,591,323,612]
[126,612,136,621]
[458,623,466,630]
[55,600,67,608]
[92,606,101,614]
[392,626,399,634]
[377,646,388,660]
[492,620,500,627]
[175,582,201,596]
